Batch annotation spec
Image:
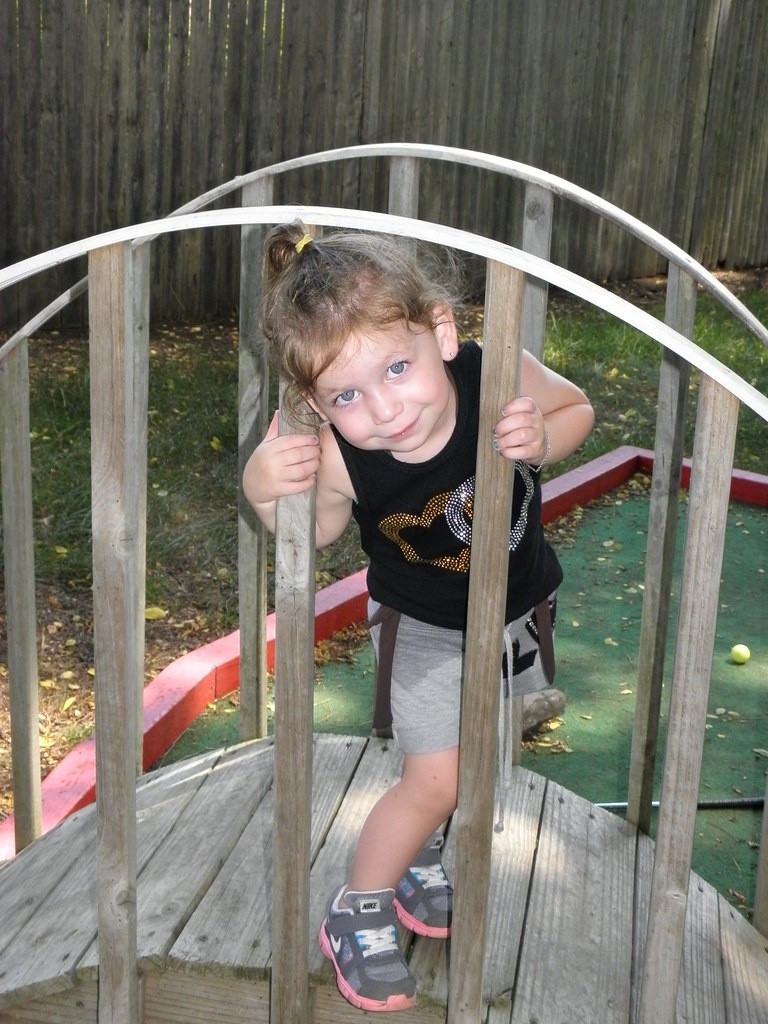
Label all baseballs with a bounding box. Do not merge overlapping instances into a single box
[730,644,751,664]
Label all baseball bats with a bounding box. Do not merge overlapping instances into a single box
[593,797,765,810]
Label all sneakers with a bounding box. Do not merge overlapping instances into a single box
[316,885,417,1012]
[392,831,454,939]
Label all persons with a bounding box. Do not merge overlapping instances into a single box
[242,219,595,1011]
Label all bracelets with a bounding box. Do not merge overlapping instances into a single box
[524,428,550,472]
